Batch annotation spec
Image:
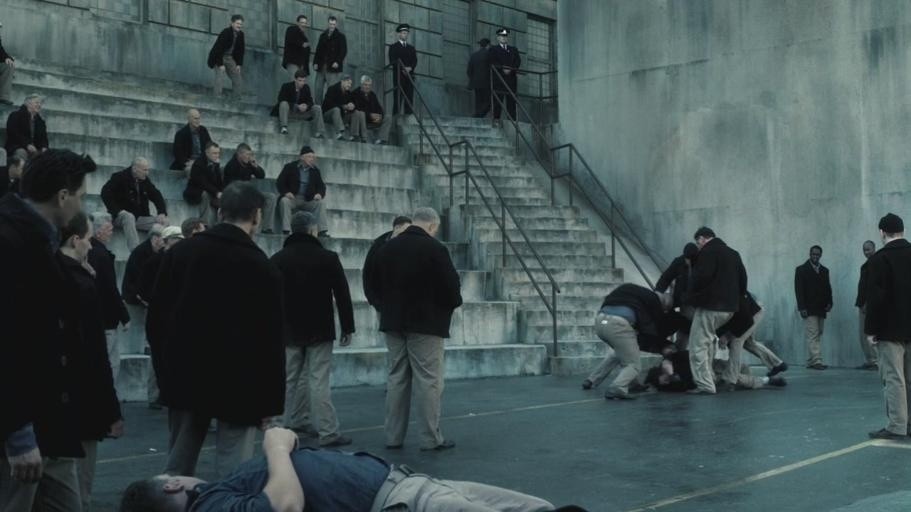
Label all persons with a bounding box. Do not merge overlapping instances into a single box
[362,216,411,276]
[866,213,911,439]
[121,427,588,512]
[854,240,879,371]
[207,14,244,97]
[794,245,833,371]
[467,38,490,117]
[487,28,521,121]
[313,17,347,105]
[388,23,418,115]
[282,15,311,82]
[364,207,463,451]
[581,227,788,400]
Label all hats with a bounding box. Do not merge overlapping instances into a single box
[396,24,410,32]
[880,213,903,233]
[161,226,186,240]
[300,146,314,155]
[496,28,511,36]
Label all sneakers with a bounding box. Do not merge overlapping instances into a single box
[869,425,910,441]
[261,229,277,235]
[856,362,879,371]
[298,423,317,435]
[320,435,353,446]
[808,364,827,370]
[440,438,456,447]
[581,377,650,401]
[685,362,789,395]
[280,127,287,133]
[318,231,331,237]
[316,133,385,145]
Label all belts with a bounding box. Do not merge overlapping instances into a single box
[371,462,415,512]
